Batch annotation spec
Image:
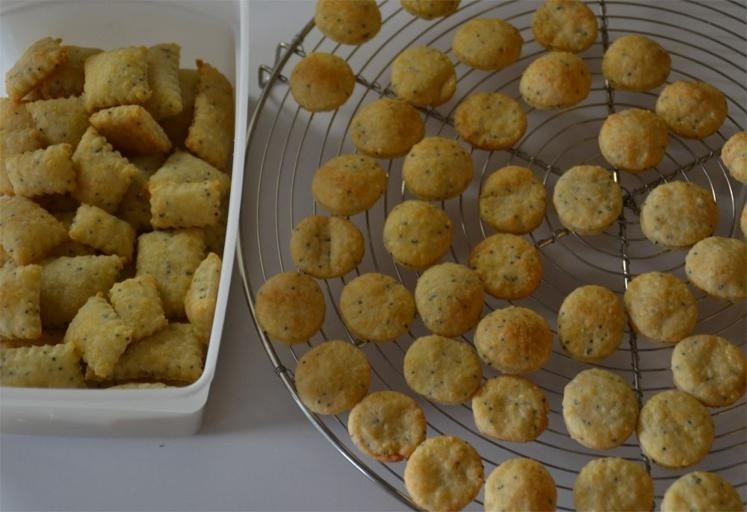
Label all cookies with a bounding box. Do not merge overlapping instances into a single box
[1,36,233,386]
[257,0,746,511]
